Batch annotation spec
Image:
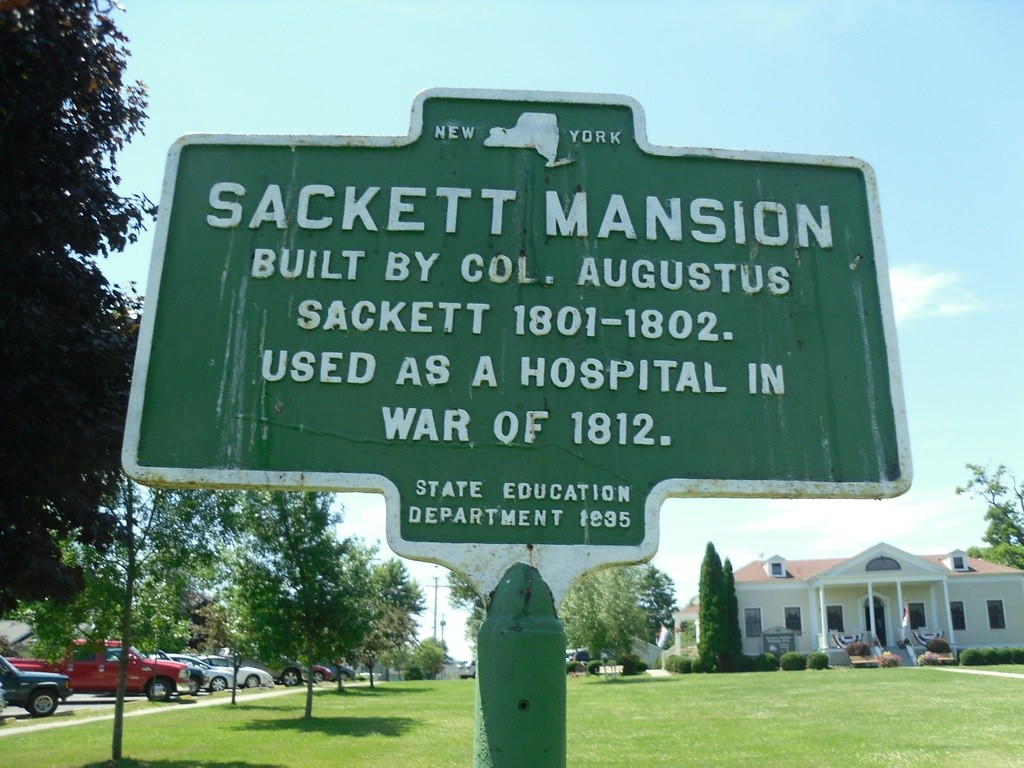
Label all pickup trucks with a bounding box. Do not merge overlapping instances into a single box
[4,638,192,703]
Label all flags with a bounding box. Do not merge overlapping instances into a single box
[902,602,911,628]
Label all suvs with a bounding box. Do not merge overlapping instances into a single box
[218,646,309,686]
[0,655,73,717]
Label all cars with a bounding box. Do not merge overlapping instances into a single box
[457,660,475,679]
[106,647,204,696]
[148,654,235,692]
[565,647,608,668]
[322,659,355,683]
[311,664,332,684]
[196,654,273,689]
